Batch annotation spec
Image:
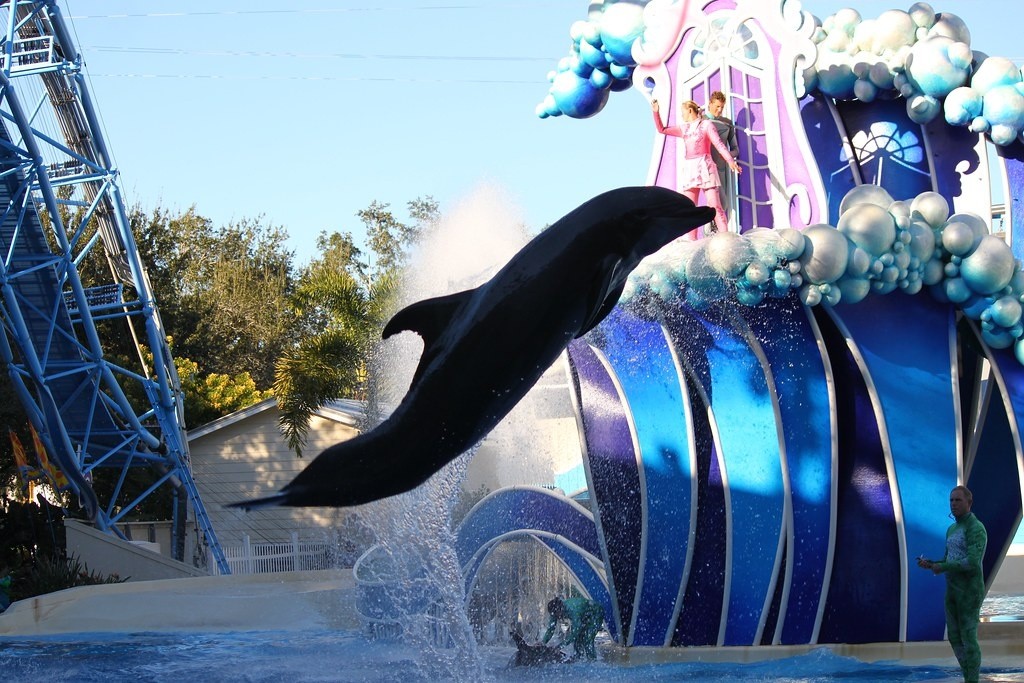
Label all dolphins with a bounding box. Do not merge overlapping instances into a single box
[504,630,577,674]
[219,184,717,516]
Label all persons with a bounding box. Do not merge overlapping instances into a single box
[533,598,605,662]
[651,98,742,241]
[701,91,739,237]
[917,486,987,683]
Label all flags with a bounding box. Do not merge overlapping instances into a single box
[10,431,46,500]
[29,421,73,499]
